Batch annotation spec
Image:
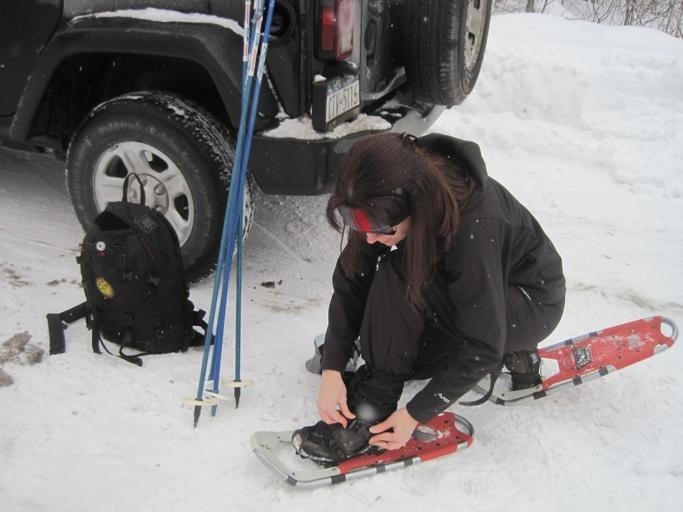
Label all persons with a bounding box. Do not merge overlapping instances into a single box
[293,128,566,464]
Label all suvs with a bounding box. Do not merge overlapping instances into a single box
[0,0,493,294]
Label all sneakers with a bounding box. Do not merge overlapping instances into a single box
[502,346,541,392]
[291,416,391,468]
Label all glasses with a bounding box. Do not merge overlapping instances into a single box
[369,224,397,235]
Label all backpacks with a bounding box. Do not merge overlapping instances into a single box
[46,173,218,367]
